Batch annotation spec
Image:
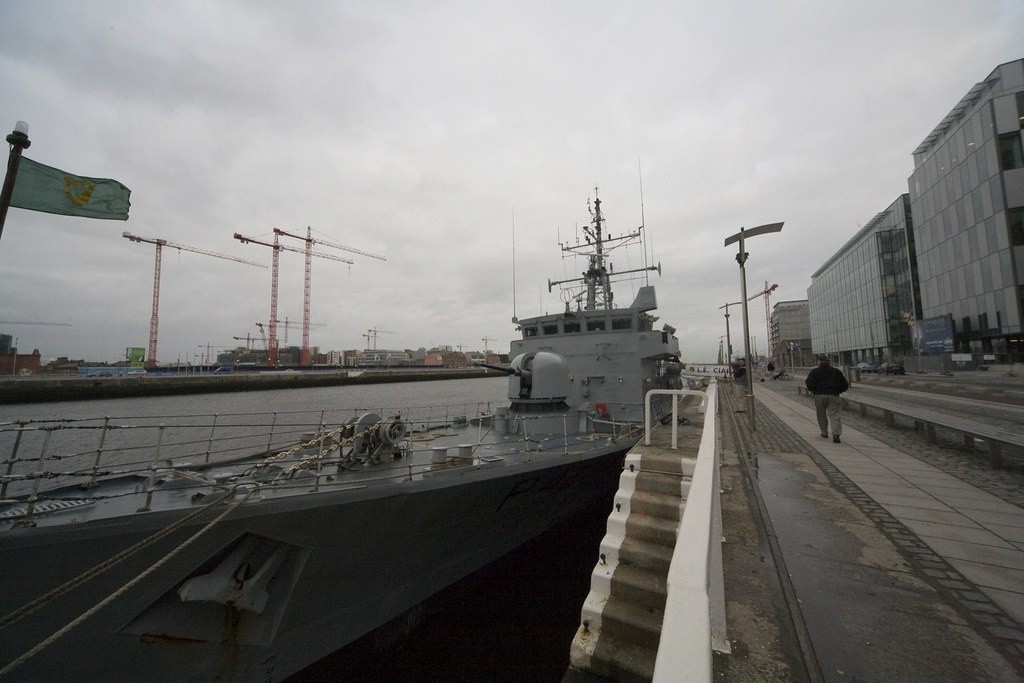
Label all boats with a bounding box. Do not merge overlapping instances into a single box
[0,146,684,683]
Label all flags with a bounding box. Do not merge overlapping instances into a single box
[8,156,132,221]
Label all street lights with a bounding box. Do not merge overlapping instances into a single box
[719,302,743,396]
[724,221,786,433]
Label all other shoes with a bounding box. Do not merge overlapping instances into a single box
[833,435,840,443]
[821,433,828,438]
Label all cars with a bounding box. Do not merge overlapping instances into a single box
[857,362,905,376]
[128,370,149,375]
[214,367,236,375]
[87,371,112,377]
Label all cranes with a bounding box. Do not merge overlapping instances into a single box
[746,281,779,366]
[123,230,269,375]
[271,225,388,368]
[234,226,353,372]
[362,327,400,350]
[482,336,496,364]
[232,316,327,353]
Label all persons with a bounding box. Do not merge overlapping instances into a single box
[687,366,697,390]
[730,358,748,413]
[767,362,775,382]
[724,373,728,382]
[805,356,848,443]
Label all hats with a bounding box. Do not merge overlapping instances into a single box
[817,355,829,361]
